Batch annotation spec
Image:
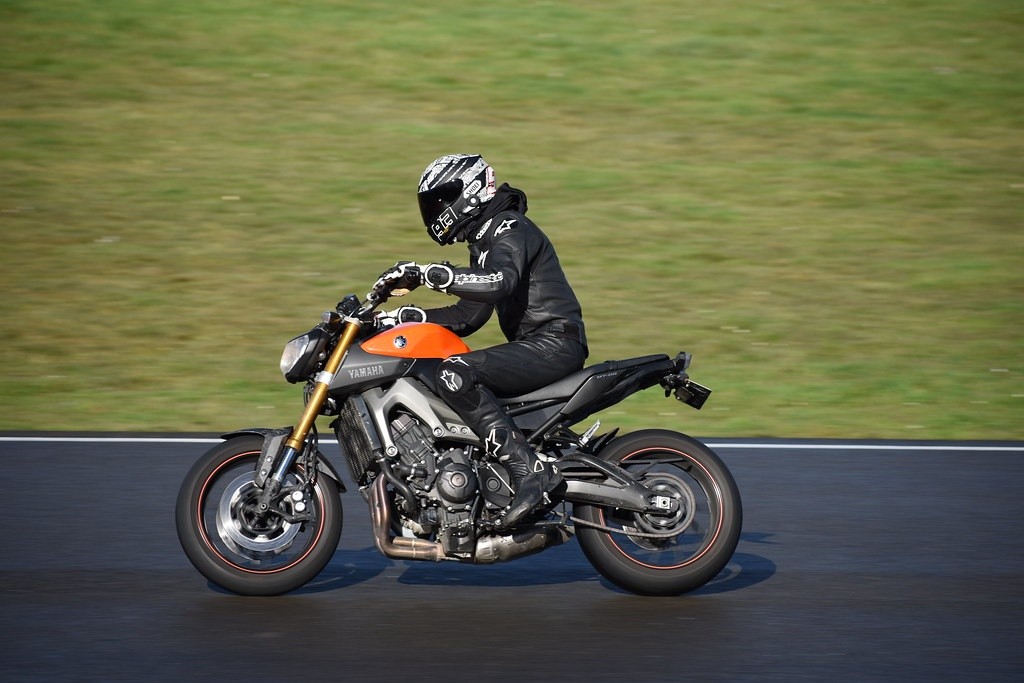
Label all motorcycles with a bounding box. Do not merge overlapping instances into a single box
[175,270,742,598]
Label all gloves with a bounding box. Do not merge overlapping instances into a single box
[374,306,400,328]
[372,260,423,301]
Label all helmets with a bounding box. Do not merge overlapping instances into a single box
[418,153,497,245]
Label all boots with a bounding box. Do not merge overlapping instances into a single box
[471,407,563,528]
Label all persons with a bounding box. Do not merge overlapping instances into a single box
[368,154,590,535]
